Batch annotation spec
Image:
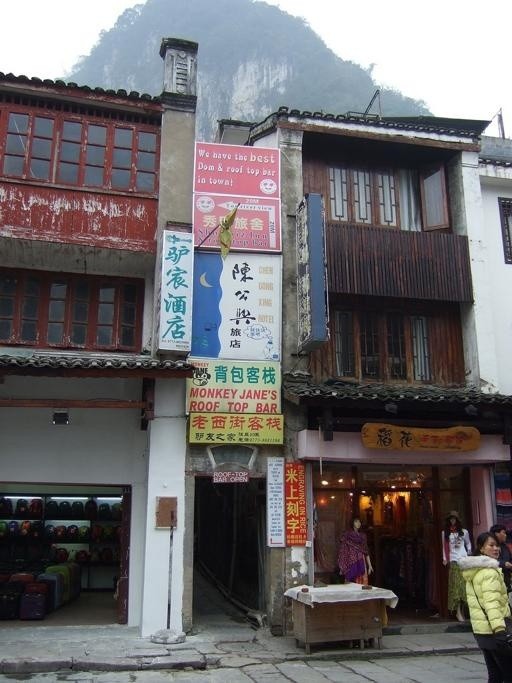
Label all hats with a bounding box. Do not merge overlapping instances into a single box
[446,510,462,522]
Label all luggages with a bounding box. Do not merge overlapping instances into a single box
[0,565,81,620]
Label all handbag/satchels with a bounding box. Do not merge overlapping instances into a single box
[494,617,512,650]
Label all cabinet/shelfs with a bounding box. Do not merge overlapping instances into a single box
[1,493,123,591]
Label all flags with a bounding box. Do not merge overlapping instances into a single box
[219,206,237,262]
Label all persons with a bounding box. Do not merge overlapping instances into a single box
[338,518,374,586]
[457,532,512,683]
[490,524,512,612]
[441,511,472,624]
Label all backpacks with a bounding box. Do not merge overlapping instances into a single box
[0,497,122,562]
